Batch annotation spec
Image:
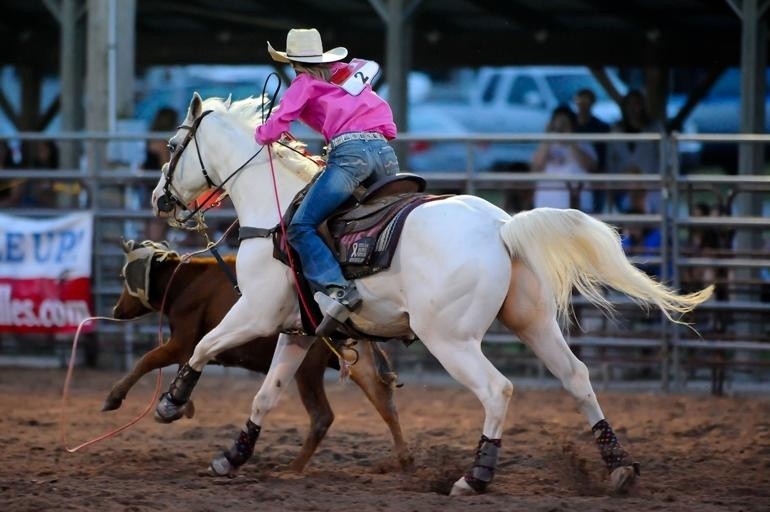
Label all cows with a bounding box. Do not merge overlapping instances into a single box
[100,236,415,475]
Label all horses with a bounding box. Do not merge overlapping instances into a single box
[151,92,714,496]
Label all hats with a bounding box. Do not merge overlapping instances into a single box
[266,26,348,65]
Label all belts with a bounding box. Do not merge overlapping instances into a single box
[326,132,387,151]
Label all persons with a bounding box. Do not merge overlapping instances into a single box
[619,208,677,291]
[253,25,400,340]
[617,162,676,215]
[688,201,713,253]
[604,87,677,214]
[574,90,610,215]
[22,140,60,208]
[709,207,732,249]
[501,161,534,215]
[0,137,26,203]
[133,107,179,243]
[528,101,599,215]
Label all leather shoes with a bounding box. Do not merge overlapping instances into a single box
[314,284,362,337]
